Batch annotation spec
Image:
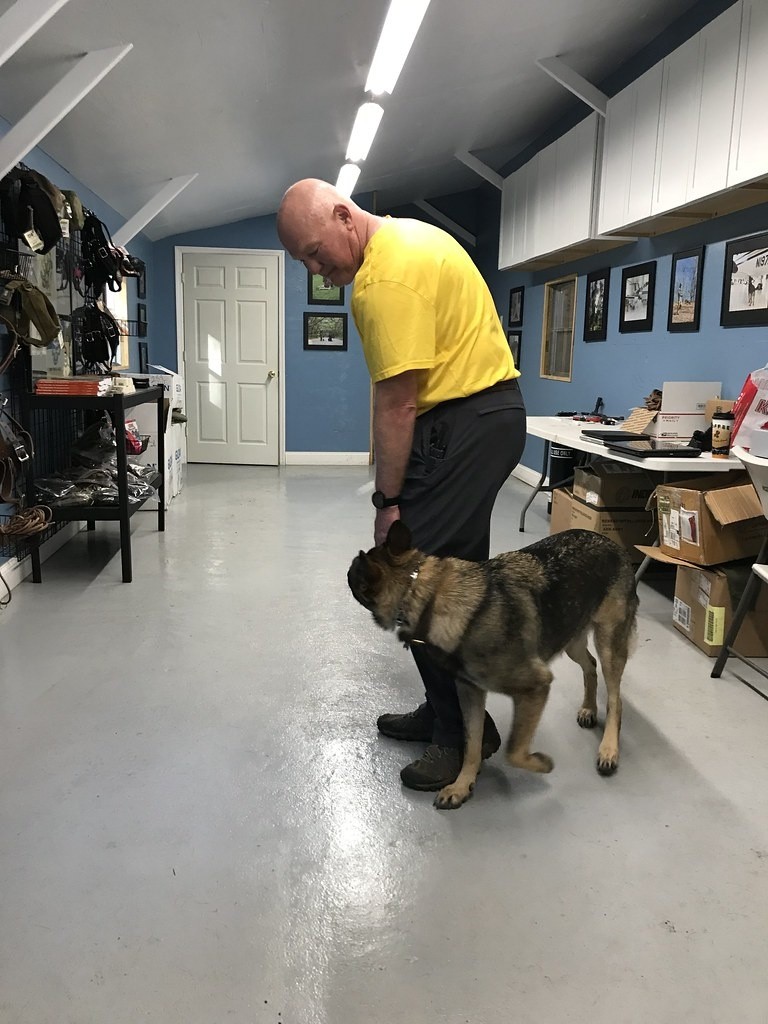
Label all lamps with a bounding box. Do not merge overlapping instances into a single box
[363,0,431,95]
[345,103,384,162]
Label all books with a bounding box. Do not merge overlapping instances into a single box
[35,378,111,396]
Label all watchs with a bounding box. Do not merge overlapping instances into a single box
[372,491,401,509]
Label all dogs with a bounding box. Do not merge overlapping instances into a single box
[349,520,640,810]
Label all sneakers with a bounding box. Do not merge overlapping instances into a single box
[400,730,501,790]
[377,708,434,742]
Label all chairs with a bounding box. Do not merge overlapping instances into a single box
[710,445,768,678]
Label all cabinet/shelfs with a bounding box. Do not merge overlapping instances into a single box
[21,161,164,583]
[498,0,768,273]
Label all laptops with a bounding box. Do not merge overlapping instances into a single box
[579,430,702,459]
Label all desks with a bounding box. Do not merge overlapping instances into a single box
[526,416,746,584]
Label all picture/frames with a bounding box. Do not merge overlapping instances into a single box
[667,245,706,331]
[138,342,149,374]
[308,269,344,306]
[720,232,768,326]
[137,264,147,299]
[303,312,348,351]
[582,267,611,341]
[508,285,525,327]
[138,303,147,337]
[619,260,657,333]
[508,330,522,370]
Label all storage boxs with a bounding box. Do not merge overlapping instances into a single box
[620,382,722,442]
[117,363,185,511]
[705,400,736,422]
[550,460,768,658]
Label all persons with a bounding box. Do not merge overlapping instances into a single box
[674,283,683,315]
[278,179,525,793]
